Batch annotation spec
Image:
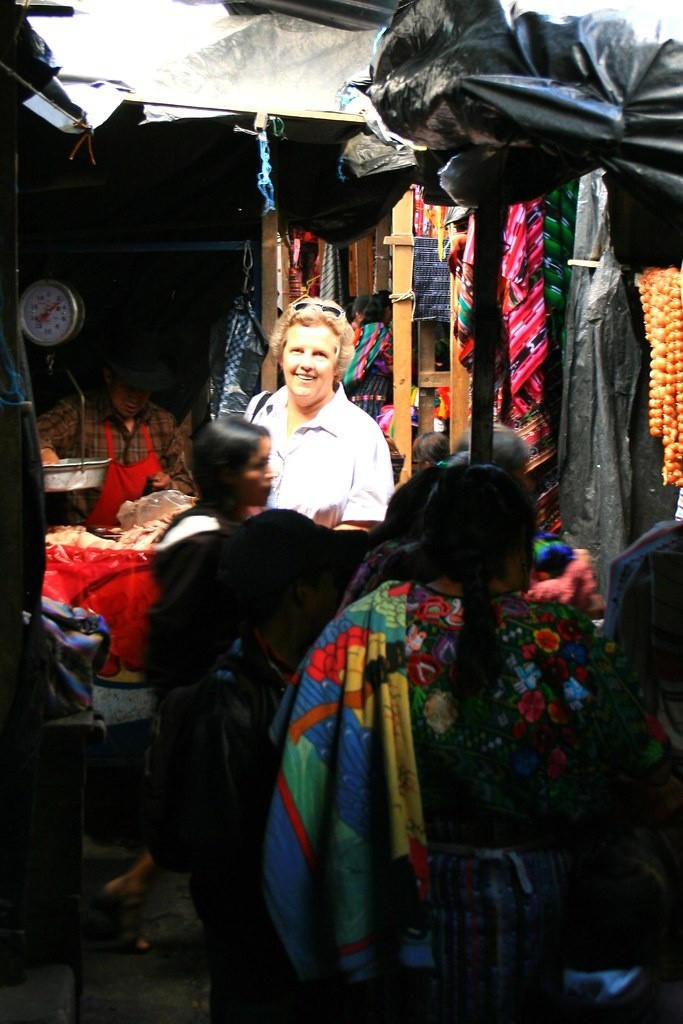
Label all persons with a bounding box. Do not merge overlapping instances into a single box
[264,431,683,1024]
[341,290,392,420]
[37,345,193,529]
[144,512,367,1024]
[102,417,275,954]
[248,297,394,529]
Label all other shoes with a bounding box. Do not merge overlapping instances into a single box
[94,882,150,953]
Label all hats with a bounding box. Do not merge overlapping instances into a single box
[103,334,177,392]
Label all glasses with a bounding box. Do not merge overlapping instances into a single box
[295,303,343,320]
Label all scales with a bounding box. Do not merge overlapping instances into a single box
[20,278,113,493]
